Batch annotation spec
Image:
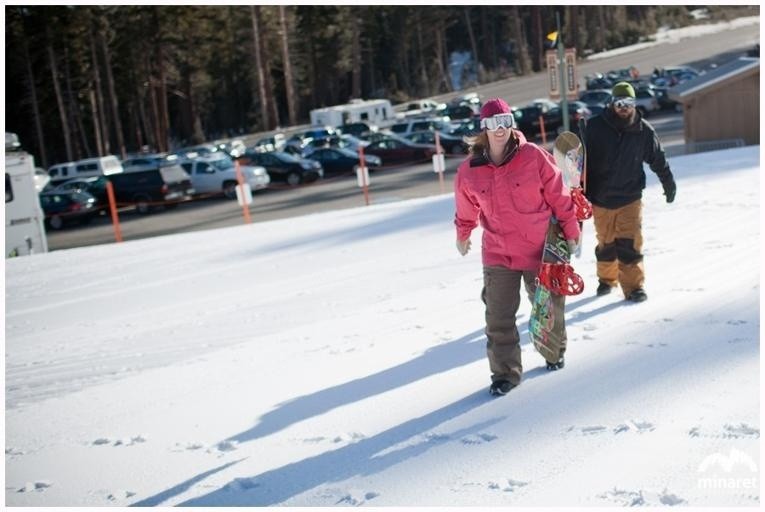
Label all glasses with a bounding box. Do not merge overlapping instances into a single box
[613,99,637,109]
[479,112,518,132]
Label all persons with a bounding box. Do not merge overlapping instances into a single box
[584,81,676,302]
[455,98,580,396]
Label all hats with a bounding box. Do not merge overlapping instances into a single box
[611,81,637,99]
[479,97,513,121]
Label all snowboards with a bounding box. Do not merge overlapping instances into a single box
[528,131,593,364]
[576,118,588,258]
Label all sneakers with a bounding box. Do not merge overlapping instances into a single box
[488,378,519,396]
[625,287,648,303]
[593,281,615,297]
[544,350,566,373]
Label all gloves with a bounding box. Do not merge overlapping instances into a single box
[453,235,473,257]
[567,236,584,260]
[663,185,677,204]
